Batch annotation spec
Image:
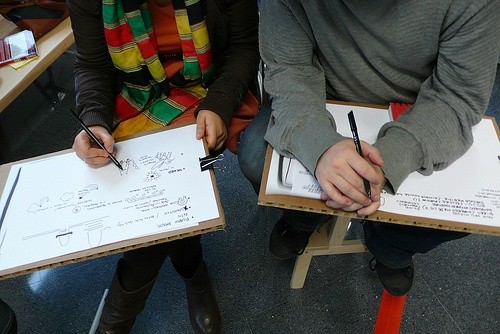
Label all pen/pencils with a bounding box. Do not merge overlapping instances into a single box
[347,110,371,198]
[66,106,124,172]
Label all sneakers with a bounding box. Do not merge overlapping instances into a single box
[269,209,333,261]
[369,257,414,296]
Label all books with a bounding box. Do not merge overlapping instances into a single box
[388,103,412,122]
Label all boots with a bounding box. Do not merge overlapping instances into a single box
[96,258,160,334]
[172,257,220,334]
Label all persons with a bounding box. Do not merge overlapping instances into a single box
[258,0,500,334]
[65,1,263,334]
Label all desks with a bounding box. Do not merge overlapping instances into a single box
[0,13,76,112]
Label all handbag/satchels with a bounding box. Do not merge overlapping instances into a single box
[223,89,261,154]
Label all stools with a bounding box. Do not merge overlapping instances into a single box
[288,215,370,290]
